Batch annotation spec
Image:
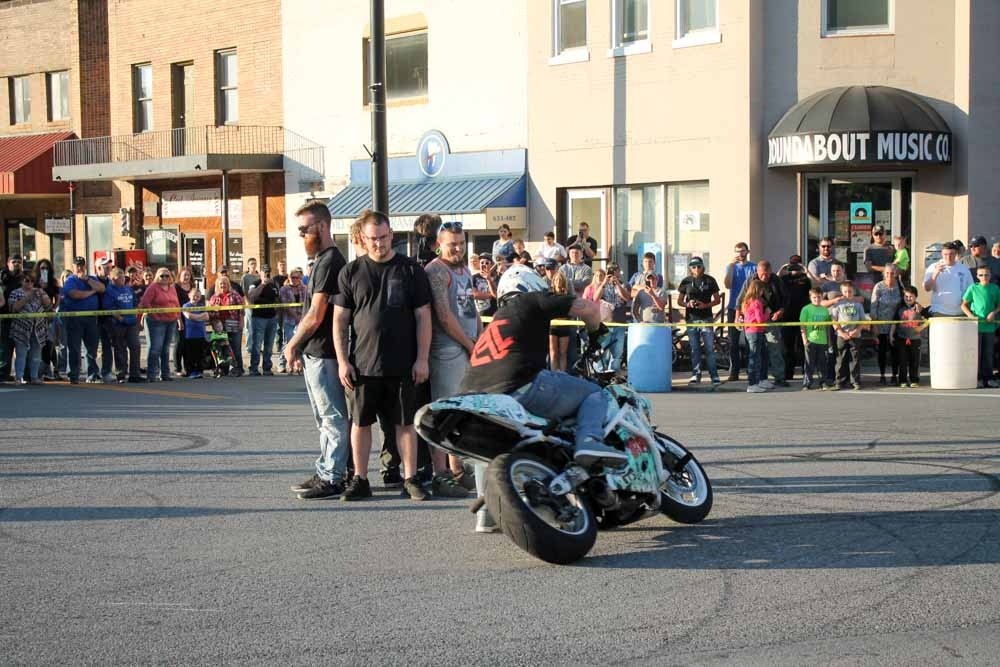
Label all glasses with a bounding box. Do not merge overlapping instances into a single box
[977,273,991,277]
[362,230,389,242]
[298,220,320,231]
[437,221,464,237]
[160,274,170,278]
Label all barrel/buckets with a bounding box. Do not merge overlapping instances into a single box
[928,317,979,390]
[627,323,672,393]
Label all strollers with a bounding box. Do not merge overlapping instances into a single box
[208,339,242,379]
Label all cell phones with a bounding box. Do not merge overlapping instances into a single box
[482,265,486,273]
[579,232,583,239]
[787,264,801,271]
[646,281,650,289]
[267,274,271,279]
[608,271,614,278]
[41,270,48,284]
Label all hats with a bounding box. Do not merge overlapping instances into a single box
[967,236,987,246]
[479,253,493,263]
[872,225,887,236]
[688,257,703,266]
[73,256,86,266]
[126,266,138,272]
[644,269,658,279]
[96,257,113,265]
[261,263,272,273]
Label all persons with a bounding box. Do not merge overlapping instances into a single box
[283,203,484,500]
[460,261,630,532]
[1,222,996,394]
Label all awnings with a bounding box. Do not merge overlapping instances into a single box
[768,85,953,169]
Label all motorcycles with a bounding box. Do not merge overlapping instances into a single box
[413,324,714,565]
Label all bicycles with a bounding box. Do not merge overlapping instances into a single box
[672,329,743,369]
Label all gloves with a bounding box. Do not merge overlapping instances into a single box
[587,322,610,347]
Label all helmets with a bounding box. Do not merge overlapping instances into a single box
[496,263,549,308]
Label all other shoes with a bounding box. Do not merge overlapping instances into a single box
[0,365,298,385]
[688,376,1000,391]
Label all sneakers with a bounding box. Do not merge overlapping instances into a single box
[291,470,477,501]
[573,438,629,469]
[477,508,501,532]
